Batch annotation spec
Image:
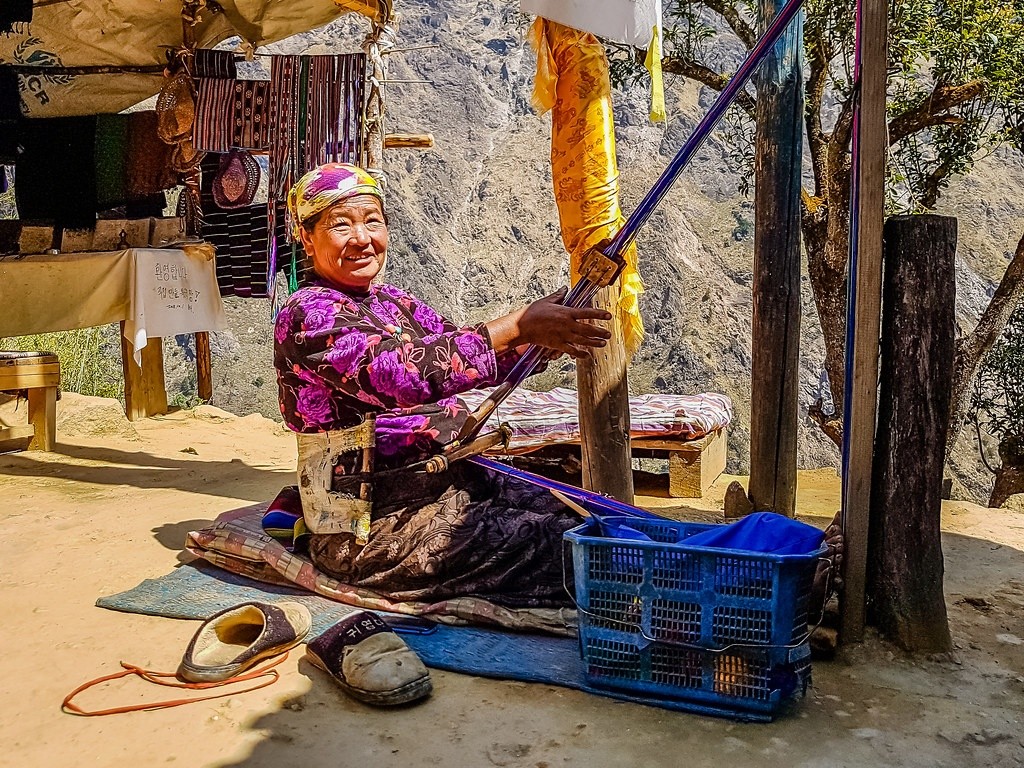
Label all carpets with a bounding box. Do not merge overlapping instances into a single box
[96,559,772,722]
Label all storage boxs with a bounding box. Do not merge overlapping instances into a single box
[562,516,829,713]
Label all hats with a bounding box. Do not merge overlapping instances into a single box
[157,74,209,173]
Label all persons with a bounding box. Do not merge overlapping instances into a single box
[274,162,844,645]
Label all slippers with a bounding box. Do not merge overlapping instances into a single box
[178,601,312,682]
[304,610,434,707]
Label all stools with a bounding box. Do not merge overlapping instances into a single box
[0,350,61,452]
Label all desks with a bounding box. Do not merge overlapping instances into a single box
[0,248,228,422]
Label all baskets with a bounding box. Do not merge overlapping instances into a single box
[562,515,830,715]
